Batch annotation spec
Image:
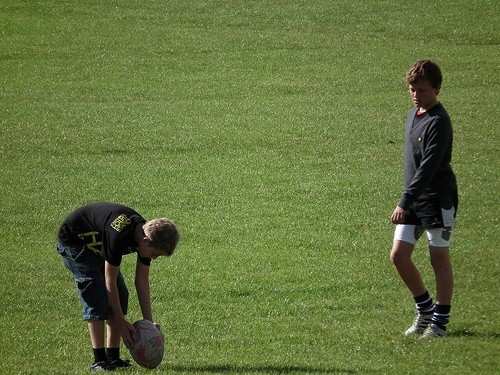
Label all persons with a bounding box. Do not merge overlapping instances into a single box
[390,59,460,340]
[56,201,180,373]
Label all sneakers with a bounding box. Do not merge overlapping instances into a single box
[403,311,433,336]
[418,324,447,340]
[91,359,132,371]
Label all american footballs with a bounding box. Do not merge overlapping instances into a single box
[128,319,164,370]
